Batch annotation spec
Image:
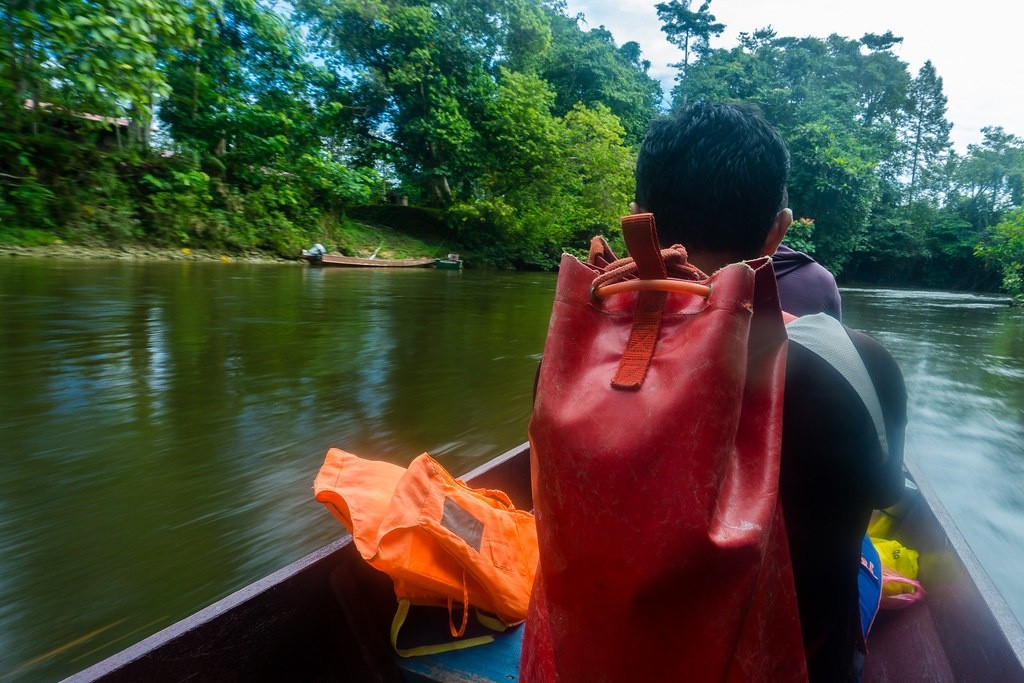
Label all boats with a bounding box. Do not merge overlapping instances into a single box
[295,254,441,268]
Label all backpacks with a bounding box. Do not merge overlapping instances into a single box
[521,210,810,682]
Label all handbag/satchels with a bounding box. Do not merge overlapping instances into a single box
[859,532,925,641]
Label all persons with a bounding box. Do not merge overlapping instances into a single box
[533,97,911,683]
[768,243,843,327]
